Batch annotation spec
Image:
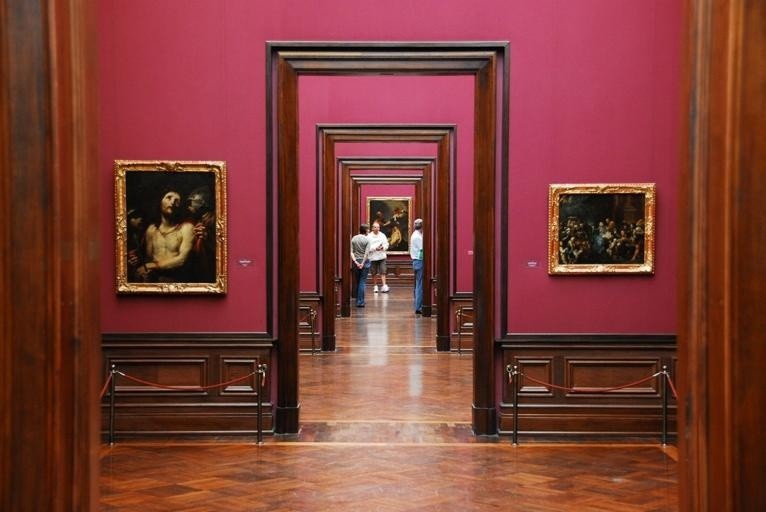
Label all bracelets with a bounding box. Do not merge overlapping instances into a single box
[144,264,148,272]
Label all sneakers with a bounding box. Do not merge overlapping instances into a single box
[373,285,389,292]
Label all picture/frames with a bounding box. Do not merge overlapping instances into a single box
[363,196,412,256]
[110,157,228,296]
[548,181,654,275]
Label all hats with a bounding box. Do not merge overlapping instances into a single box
[414,219,422,227]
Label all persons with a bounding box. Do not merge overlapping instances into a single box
[410,218,424,314]
[186,188,215,282]
[367,222,390,292]
[127,190,195,283]
[128,209,146,282]
[559,216,644,264]
[350,224,371,307]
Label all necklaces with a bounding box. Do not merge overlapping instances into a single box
[158,224,181,238]
